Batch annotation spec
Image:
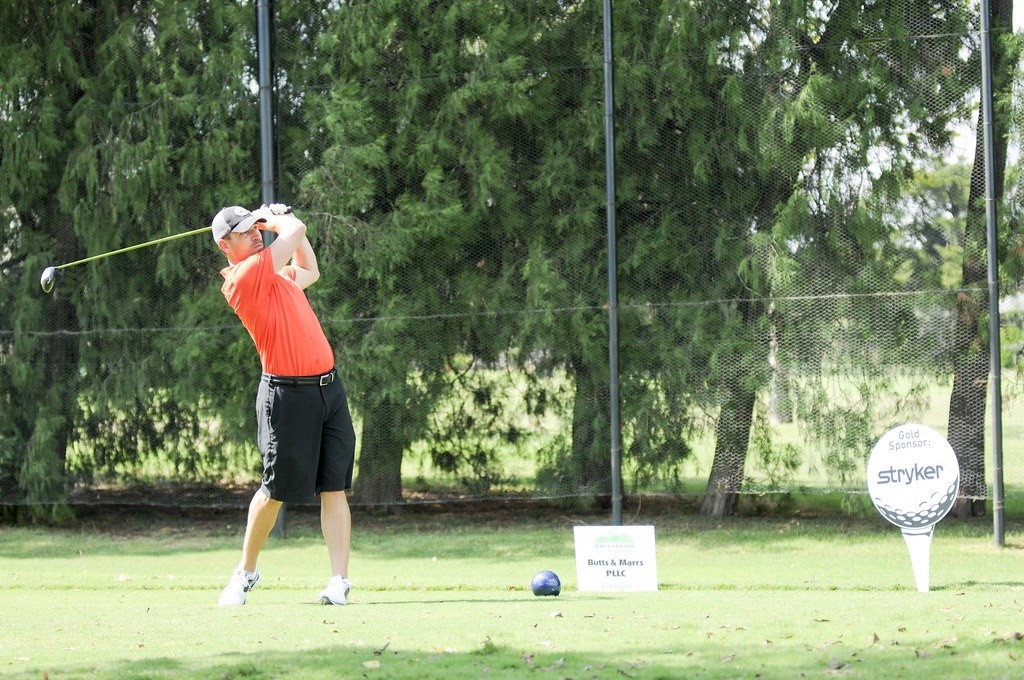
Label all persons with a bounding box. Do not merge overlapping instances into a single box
[212,203,356,605]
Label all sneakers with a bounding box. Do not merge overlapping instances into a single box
[218,561,260,605]
[321,575,352,606]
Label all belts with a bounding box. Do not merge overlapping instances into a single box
[262,370,338,386]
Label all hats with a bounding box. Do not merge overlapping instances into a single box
[211,205,267,244]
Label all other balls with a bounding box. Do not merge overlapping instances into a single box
[531,570,561,596]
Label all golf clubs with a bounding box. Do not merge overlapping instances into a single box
[40,206,292,293]
